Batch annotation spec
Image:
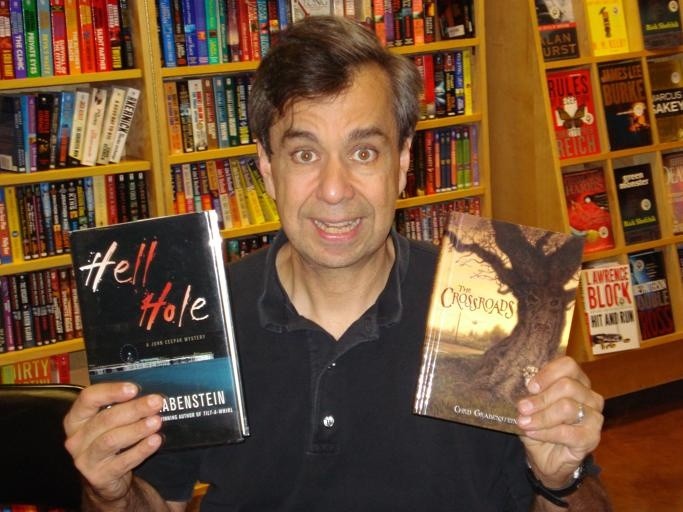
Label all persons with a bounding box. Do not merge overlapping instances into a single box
[61,10,614,511]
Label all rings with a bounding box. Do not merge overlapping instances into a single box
[576,402,583,424]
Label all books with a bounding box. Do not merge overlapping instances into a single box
[157,2,483,264]
[1,86,151,354]
[411,211,586,438]
[1,1,134,80]
[534,2,682,353]
[66,210,251,450]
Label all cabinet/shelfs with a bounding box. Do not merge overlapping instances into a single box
[0,1,683,404]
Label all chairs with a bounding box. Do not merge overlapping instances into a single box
[0,380,86,510]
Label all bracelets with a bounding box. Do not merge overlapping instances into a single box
[521,456,587,508]
